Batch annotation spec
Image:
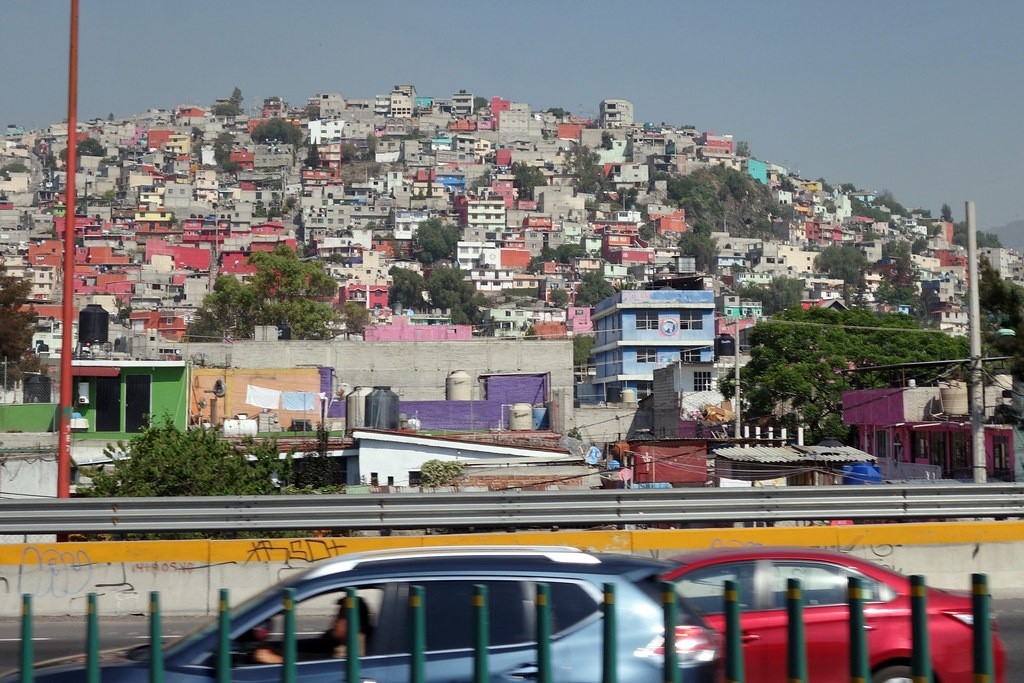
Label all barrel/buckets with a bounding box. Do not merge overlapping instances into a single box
[622,388,634,402]
[446,370,472,401]
[842,462,882,485]
[347,387,373,437]
[532,408,548,430]
[79,304,109,342]
[940,387,968,415]
[364,387,399,431]
[23,370,51,403]
[508,403,533,431]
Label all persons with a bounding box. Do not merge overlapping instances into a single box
[254,596,372,664]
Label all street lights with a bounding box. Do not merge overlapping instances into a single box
[967,328,1016,485]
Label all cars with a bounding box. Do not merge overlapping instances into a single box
[638,547,1005,683]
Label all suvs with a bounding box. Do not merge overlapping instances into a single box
[18,546,722,682]
